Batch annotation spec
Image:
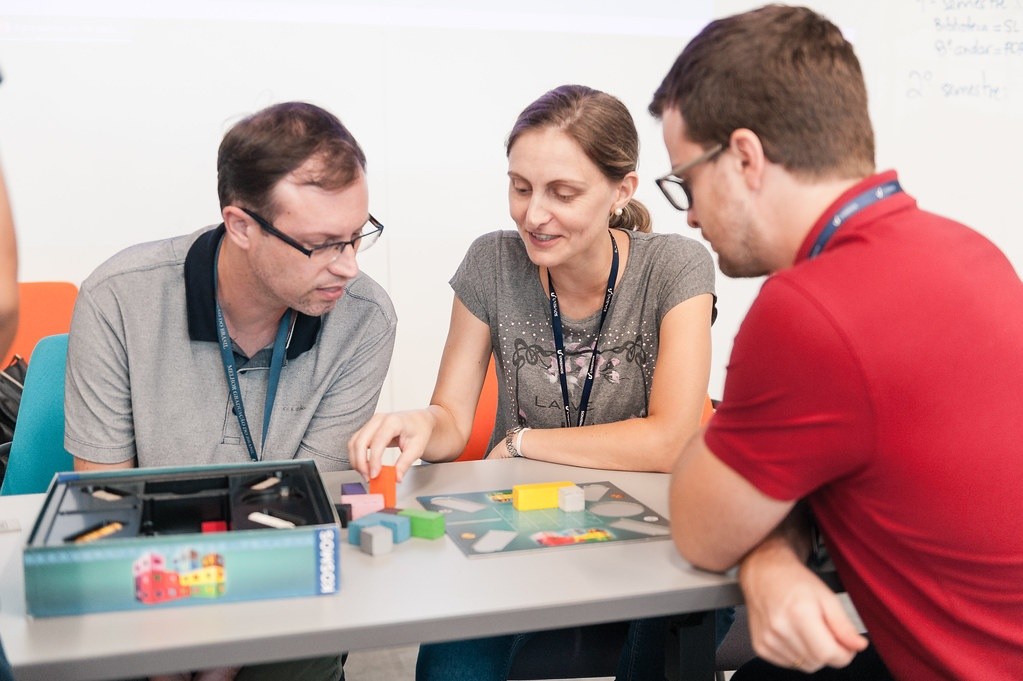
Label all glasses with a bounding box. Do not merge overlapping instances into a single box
[240,207,384,264]
[656,142,731,211]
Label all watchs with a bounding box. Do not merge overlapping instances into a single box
[505,426,522,457]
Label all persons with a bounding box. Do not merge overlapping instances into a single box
[0,69,20,681]
[648,3,1022,680]
[347,85,719,681]
[62,101,398,681]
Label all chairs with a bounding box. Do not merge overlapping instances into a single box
[451,352,715,462]
[0,335,75,496]
[0,282,79,371]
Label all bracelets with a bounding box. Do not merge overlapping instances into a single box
[516,427,531,457]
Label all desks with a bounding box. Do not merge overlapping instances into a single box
[0,458,844,681]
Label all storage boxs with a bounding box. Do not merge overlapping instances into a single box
[22,458,342,620]
